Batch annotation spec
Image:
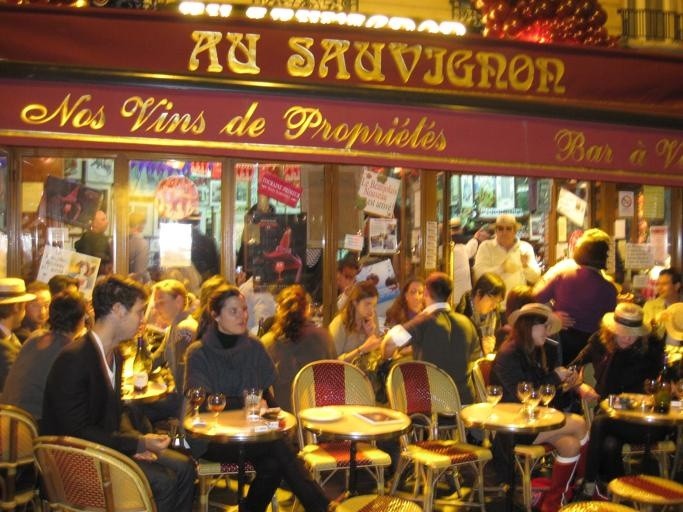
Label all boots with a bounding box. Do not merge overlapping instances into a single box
[566,431,589,500]
[539,457,580,512]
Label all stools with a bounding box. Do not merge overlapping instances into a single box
[335,493,421,512]
[607,475,682,512]
[191,454,277,511]
[558,500,638,512]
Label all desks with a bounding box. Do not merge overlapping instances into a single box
[297,403,413,498]
[181,406,298,511]
[461,401,566,512]
[120,377,168,411]
[600,394,683,473]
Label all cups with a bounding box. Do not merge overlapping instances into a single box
[517,383,532,416]
[675,382,683,413]
[486,386,502,419]
[525,393,541,424]
[538,384,555,419]
[243,388,263,427]
[207,393,226,429]
[644,379,661,407]
[188,388,206,427]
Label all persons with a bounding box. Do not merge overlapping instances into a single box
[475,213,540,300]
[645,268,683,325]
[531,228,636,301]
[0,276,41,395]
[330,281,392,404]
[573,302,664,498]
[74,209,112,276]
[46,272,85,331]
[497,284,541,351]
[543,228,618,361]
[258,283,340,442]
[491,303,591,512]
[450,216,462,236]
[149,279,200,423]
[379,271,483,500]
[127,212,150,277]
[316,257,363,304]
[457,272,505,360]
[26,286,52,338]
[181,284,337,512]
[37,276,195,512]
[2,292,84,421]
[384,277,426,337]
[185,209,221,278]
[438,227,490,306]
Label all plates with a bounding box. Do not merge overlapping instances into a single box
[299,407,345,422]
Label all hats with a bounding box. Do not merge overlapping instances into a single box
[664,302,683,341]
[0,277,37,304]
[603,302,652,337]
[488,214,522,230]
[508,302,561,336]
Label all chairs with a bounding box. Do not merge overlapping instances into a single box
[386,359,492,512]
[579,362,677,478]
[290,359,392,511]
[31,434,157,512]
[466,357,556,512]
[0,403,40,512]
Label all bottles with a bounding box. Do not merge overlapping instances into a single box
[134,335,149,392]
[654,354,671,414]
